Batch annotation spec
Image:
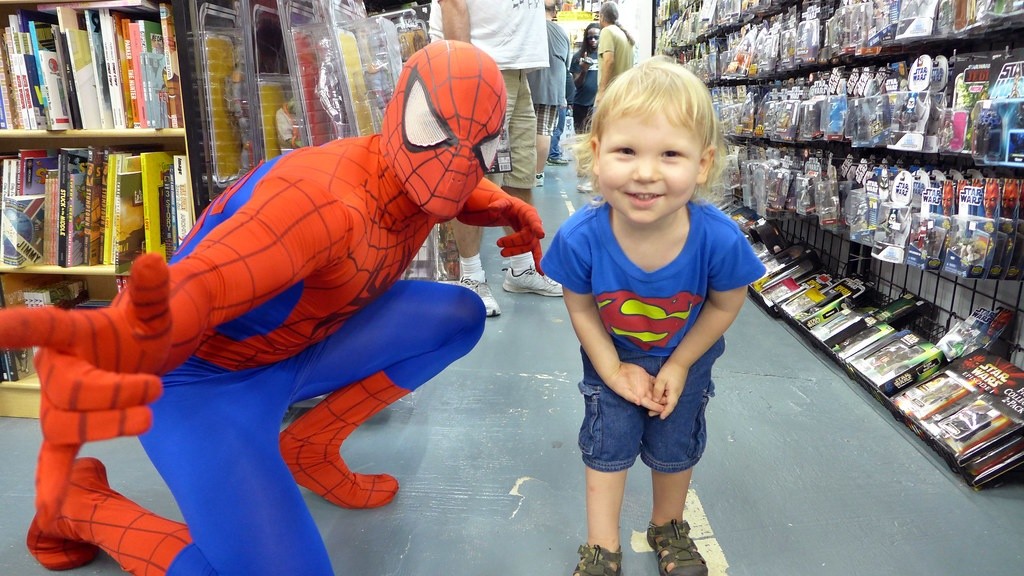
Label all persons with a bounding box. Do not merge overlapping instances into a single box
[429,0,564,318]
[537,52,767,576]
[526,0,635,193]
[217,23,395,179]
[0,39,545,576]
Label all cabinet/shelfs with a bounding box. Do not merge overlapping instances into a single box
[0,0,208,418]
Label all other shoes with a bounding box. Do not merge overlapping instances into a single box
[535,172,545,187]
[546,157,569,166]
[577,181,594,193]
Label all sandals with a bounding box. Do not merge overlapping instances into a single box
[647,519,708,576]
[573,543,622,576]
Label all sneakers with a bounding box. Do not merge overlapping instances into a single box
[459,275,501,316]
[502,263,564,297]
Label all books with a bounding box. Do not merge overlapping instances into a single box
[0,0,197,381]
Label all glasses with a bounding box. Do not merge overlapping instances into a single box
[587,35,600,40]
